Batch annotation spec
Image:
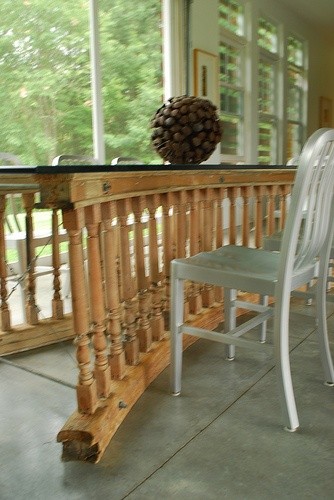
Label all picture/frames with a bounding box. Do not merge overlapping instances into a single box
[191,47,220,109]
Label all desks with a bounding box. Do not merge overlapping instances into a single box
[2,161,334,465]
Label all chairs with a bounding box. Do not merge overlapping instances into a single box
[167,124,334,434]
